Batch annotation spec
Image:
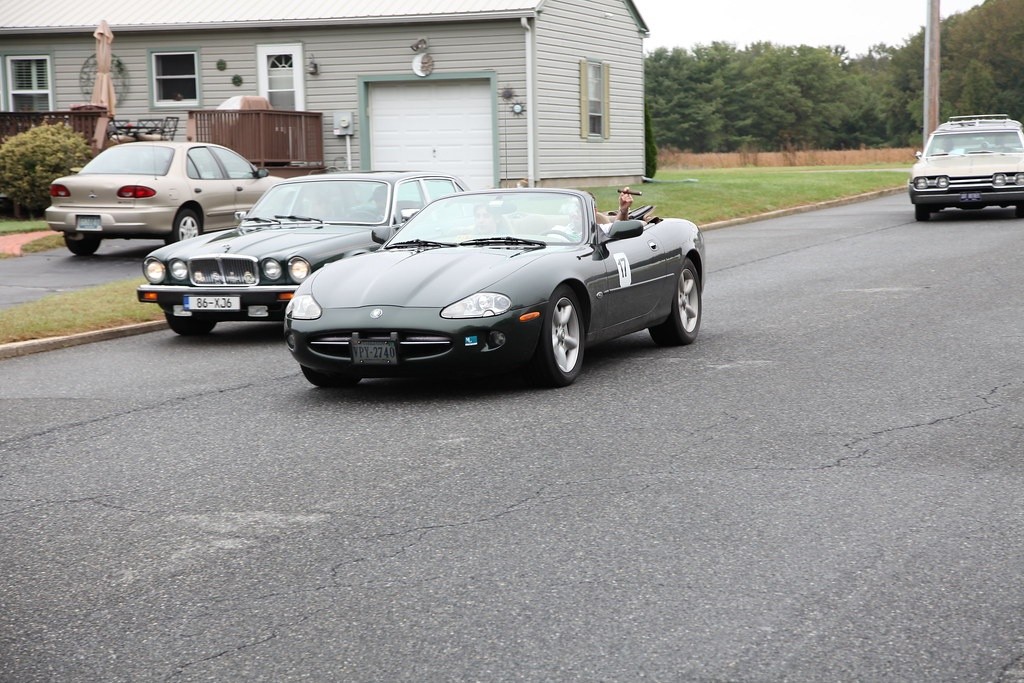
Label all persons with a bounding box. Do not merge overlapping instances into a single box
[472,203,508,236]
[995,134,1005,146]
[548,187,634,241]
[943,138,954,152]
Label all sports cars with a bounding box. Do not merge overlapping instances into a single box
[284,186,705,389]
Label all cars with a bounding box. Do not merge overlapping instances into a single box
[137,171,472,334]
[908,113,1024,222]
[45,141,286,256]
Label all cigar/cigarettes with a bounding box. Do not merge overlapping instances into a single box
[618,189,642,195]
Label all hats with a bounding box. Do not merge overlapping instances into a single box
[369,186,387,202]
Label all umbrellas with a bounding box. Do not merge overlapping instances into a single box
[90,20,118,116]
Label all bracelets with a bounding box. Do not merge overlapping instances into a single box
[619,207,628,214]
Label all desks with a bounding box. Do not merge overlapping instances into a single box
[116,126,162,142]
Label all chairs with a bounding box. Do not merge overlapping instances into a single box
[111,117,179,142]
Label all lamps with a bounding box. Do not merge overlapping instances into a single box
[411,37,428,52]
